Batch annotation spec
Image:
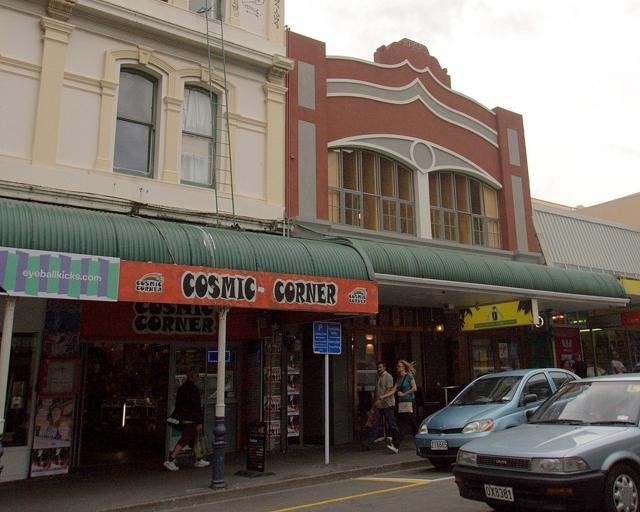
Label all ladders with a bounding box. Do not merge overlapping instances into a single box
[203,0,236,228]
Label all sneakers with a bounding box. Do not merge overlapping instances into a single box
[164,461,179,471]
[374,436,391,442]
[387,444,399,453]
[195,460,210,467]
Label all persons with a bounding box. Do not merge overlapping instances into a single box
[378,358,421,453]
[361,361,402,449]
[163,366,213,472]
[559,350,640,379]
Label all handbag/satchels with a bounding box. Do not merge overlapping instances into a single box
[398,402,413,413]
[168,409,183,425]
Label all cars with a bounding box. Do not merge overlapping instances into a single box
[414,368,583,470]
[453,373,639,511]
[355,368,377,399]
